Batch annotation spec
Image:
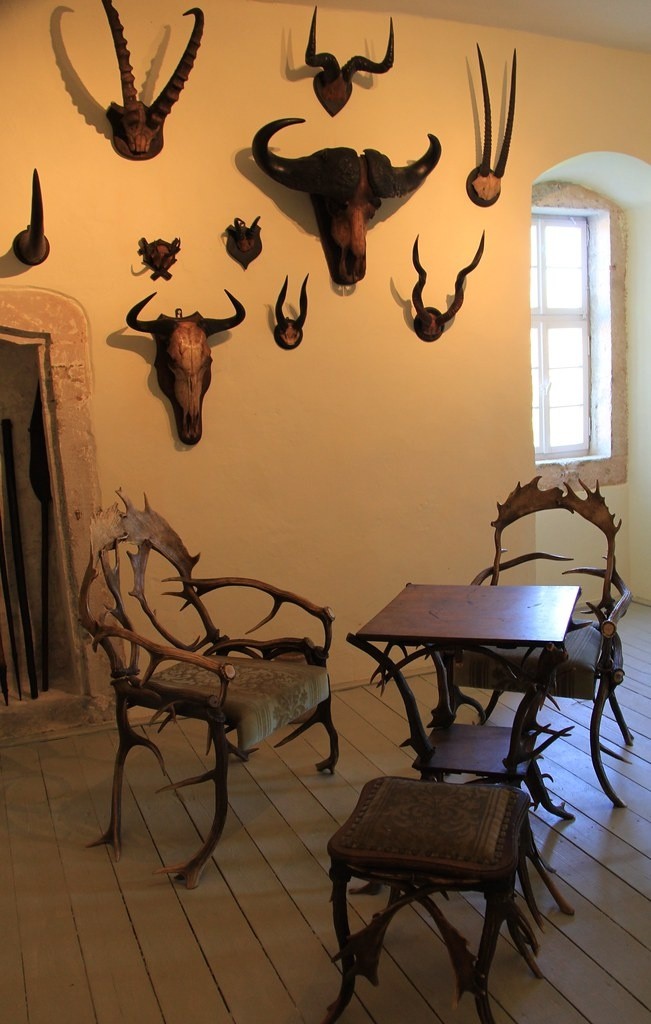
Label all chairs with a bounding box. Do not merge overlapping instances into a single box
[74,489,338,891]
[413,477,637,810]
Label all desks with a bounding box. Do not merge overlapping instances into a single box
[346,583,581,822]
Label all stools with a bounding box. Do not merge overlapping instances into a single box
[323,775,575,1024]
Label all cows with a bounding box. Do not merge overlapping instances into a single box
[252,118,442,287]
[127,288,245,446]
